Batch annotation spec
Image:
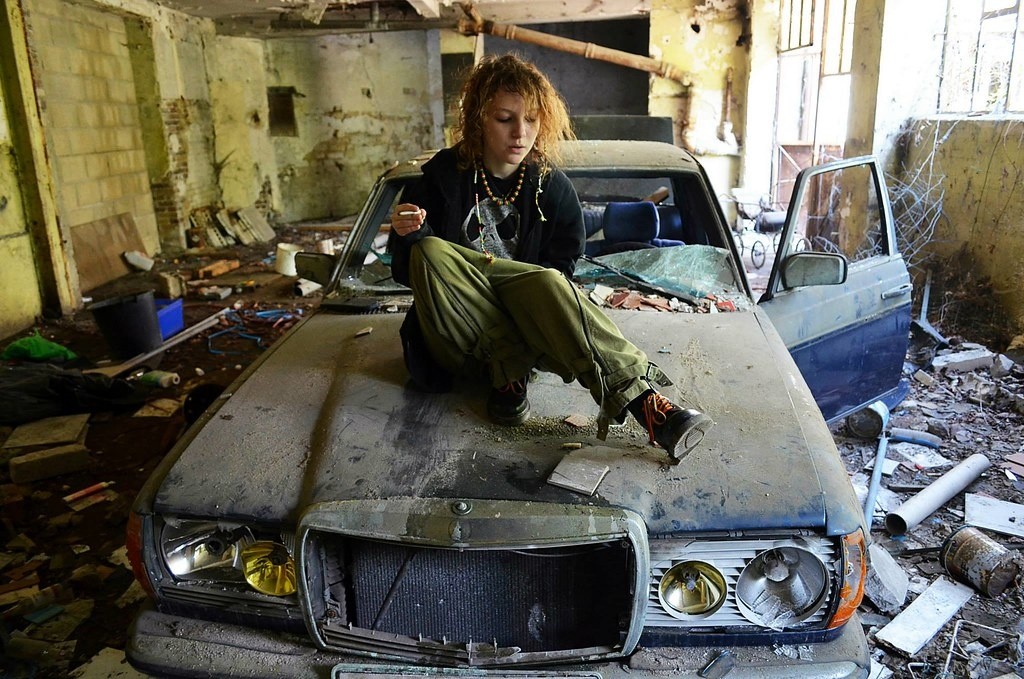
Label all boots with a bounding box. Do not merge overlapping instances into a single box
[628,379,714,465]
[488,374,531,426]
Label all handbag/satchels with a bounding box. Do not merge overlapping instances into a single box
[0,327,77,364]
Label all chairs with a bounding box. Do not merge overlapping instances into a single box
[584,203,686,255]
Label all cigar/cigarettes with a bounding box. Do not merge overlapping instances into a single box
[399,211,421,215]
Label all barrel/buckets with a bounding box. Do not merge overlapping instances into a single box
[940,525,1024,599]
[275,243,304,277]
[88,288,163,370]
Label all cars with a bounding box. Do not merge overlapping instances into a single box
[125,136,912,679]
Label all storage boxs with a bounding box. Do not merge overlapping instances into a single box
[156,298,185,340]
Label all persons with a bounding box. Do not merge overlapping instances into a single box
[386,56,714,459]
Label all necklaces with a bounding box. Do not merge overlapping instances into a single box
[479,160,525,205]
